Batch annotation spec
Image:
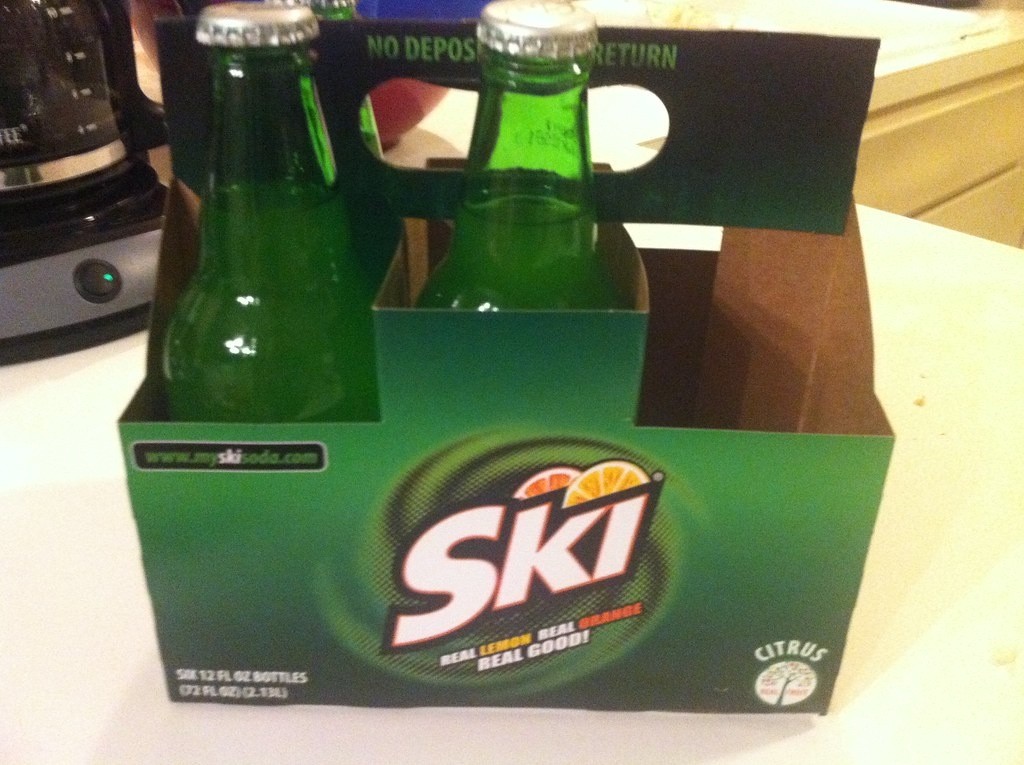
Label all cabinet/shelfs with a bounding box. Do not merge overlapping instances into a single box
[850,48,1024,249]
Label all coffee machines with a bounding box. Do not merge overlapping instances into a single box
[0,1,197,352]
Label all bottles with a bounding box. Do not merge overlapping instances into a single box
[265,0,399,280]
[165,2,384,421]
[415,1,628,311]
